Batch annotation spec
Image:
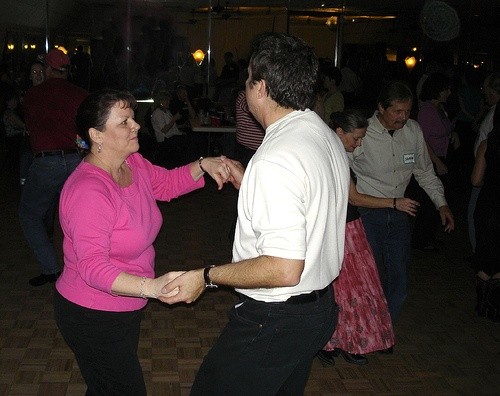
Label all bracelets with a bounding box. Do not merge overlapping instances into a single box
[393,198,397,210]
[199,157,207,173]
[138,276,146,300]
[204,265,217,290]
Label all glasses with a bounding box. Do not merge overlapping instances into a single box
[30,69,43,73]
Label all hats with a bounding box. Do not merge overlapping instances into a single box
[38,49,71,72]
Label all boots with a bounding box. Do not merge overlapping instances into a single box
[475,276,500,322]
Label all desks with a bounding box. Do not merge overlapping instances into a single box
[194,124,236,158]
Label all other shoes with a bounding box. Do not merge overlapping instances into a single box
[315,349,368,367]
[414,239,445,251]
[463,256,476,269]
[29,272,61,286]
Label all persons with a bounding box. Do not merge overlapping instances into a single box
[14,48,90,286]
[157,33,349,396]
[53,87,232,396]
[0,45,90,192]
[146,42,500,365]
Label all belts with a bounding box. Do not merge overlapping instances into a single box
[285,286,328,304]
[32,147,80,159]
